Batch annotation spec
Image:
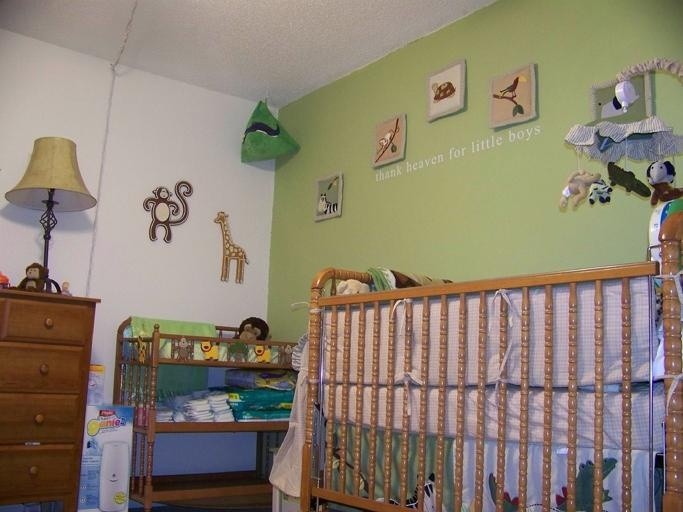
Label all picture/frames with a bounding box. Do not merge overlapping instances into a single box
[488,63,538,127]
[371,113,406,167]
[425,58,466,121]
[312,171,342,221]
[590,71,652,121]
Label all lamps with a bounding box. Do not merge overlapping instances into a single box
[5,136,98,294]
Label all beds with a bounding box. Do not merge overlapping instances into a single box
[113,316,298,512]
[300,211,683,512]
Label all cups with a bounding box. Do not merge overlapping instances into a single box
[99,441,130,512]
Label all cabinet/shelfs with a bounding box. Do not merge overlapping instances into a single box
[0,288,101,512]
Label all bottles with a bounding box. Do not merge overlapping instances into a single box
[78,440,102,510]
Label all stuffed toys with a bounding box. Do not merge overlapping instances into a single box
[650,182,682,206]
[647,160,677,185]
[607,161,652,197]
[560,170,602,207]
[233,317,269,341]
[336,278,370,307]
[9,262,49,292]
[587,178,613,206]
